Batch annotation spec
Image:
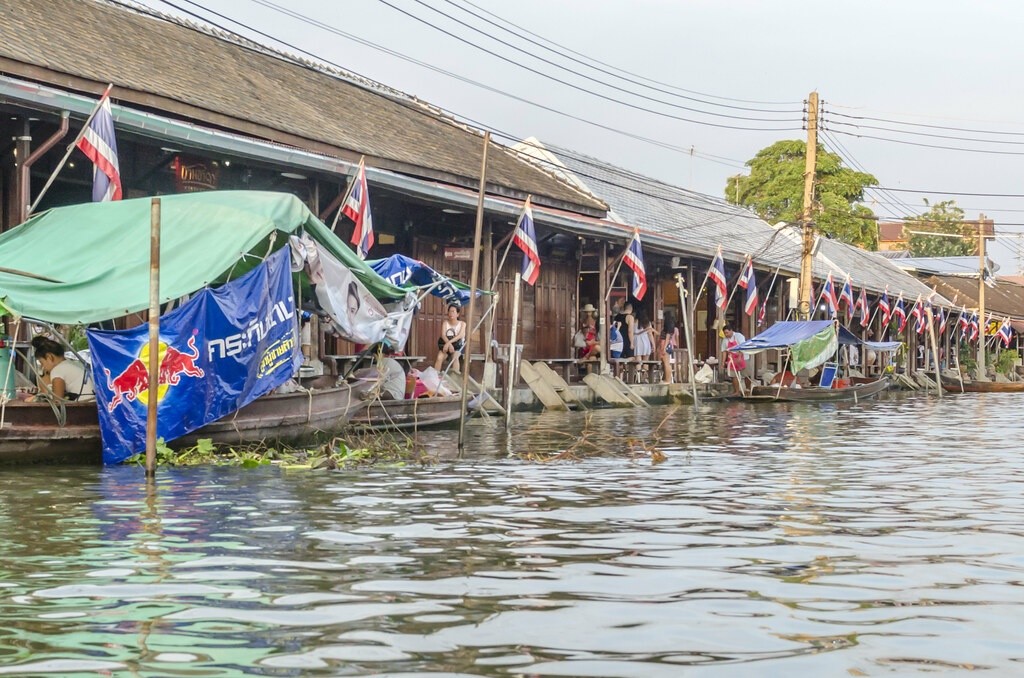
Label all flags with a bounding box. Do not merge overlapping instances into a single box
[821,276,839,313]
[512,202,541,287]
[857,289,936,334]
[73,91,123,202]
[936,306,1013,349]
[341,161,374,258]
[737,261,758,316]
[841,277,853,318]
[622,229,647,302]
[706,252,728,309]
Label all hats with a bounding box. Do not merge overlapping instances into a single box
[582,303,595,311]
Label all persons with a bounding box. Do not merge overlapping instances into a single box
[720,325,750,395]
[917,340,924,367]
[32,336,97,404]
[435,303,467,372]
[371,346,406,400]
[302,321,311,359]
[347,281,359,324]
[579,298,676,385]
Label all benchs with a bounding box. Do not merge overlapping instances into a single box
[322,355,427,377]
[529,358,720,383]
[457,354,486,374]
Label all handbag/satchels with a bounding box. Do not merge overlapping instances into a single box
[438,328,462,351]
[666,344,673,354]
[694,364,714,383]
[573,329,587,348]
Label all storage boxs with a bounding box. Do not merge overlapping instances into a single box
[830,378,850,389]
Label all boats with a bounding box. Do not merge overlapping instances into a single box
[726,320,904,402]
[0,190,502,465]
[942,380,1024,392]
